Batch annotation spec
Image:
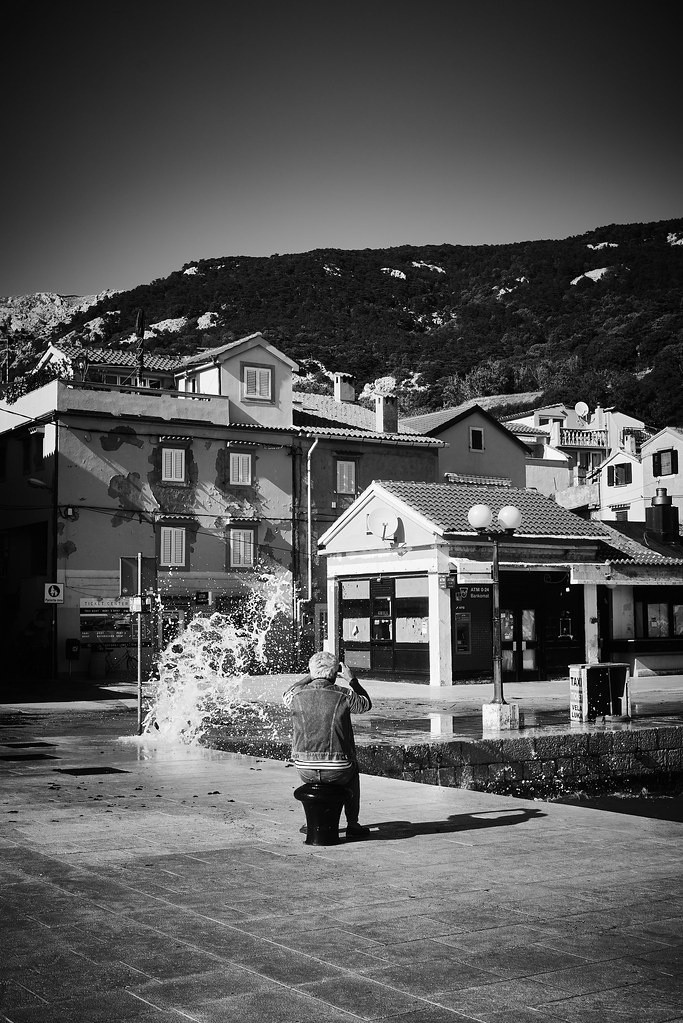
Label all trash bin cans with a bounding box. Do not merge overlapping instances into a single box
[569,663,630,721]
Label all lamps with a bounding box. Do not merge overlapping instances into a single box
[196,591,208,605]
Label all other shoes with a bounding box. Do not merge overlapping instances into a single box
[346,823,370,838]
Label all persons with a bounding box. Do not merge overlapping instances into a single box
[281,652,373,840]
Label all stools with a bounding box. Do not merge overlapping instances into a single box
[293,782,344,847]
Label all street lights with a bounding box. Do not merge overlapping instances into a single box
[468,503,525,733]
